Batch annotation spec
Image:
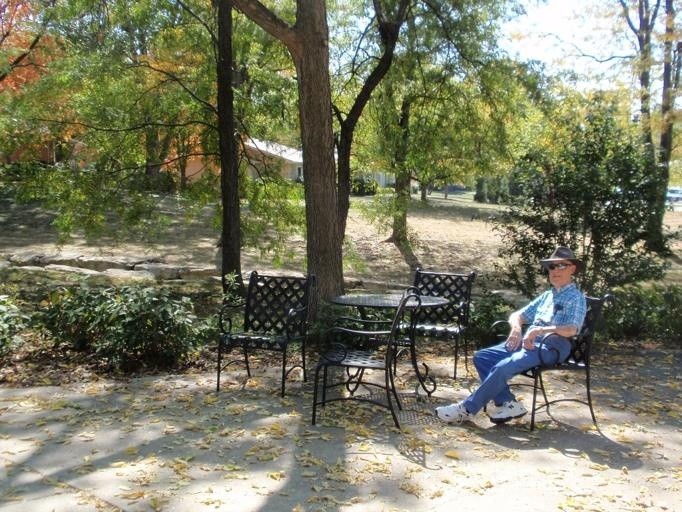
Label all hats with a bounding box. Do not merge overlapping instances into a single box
[539,246,582,274]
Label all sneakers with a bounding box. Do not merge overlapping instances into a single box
[490,399,528,424]
[435,400,470,427]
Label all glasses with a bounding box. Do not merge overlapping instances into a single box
[548,262,576,271]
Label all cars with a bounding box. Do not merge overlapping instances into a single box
[666,189,682,202]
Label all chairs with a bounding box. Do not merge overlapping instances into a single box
[392,268,477,381]
[311,286,422,428]
[216,270,316,398]
[482,268,610,430]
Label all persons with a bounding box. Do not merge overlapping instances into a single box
[434,245,588,425]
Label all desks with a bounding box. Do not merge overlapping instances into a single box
[328,291,450,395]
